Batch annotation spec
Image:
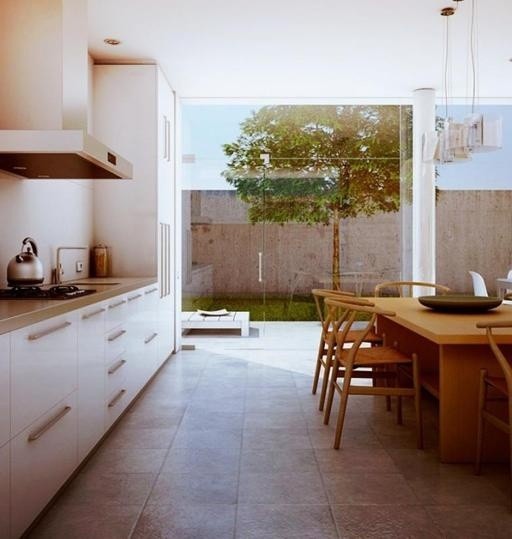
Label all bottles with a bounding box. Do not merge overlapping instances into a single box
[95,242,107,278]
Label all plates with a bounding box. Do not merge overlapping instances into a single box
[419,295,502,312]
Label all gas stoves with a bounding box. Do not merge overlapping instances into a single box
[0,286,98,301]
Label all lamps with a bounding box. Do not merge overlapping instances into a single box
[424,7,471,165]
[462,1,503,153]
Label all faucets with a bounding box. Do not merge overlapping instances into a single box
[55,246,87,284]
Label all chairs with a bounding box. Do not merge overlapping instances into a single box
[497,279,512,302]
[324,298,424,451]
[474,322,512,475]
[313,287,390,412]
[375,281,448,297]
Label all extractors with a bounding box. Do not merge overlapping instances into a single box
[0,126,132,181]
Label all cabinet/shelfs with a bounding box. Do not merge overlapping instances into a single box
[79,293,128,466]
[94,61,175,368]
[126,281,158,409]
[0,308,80,539]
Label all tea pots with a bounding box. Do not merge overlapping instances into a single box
[6,238,44,285]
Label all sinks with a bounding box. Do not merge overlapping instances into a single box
[66,283,121,291]
